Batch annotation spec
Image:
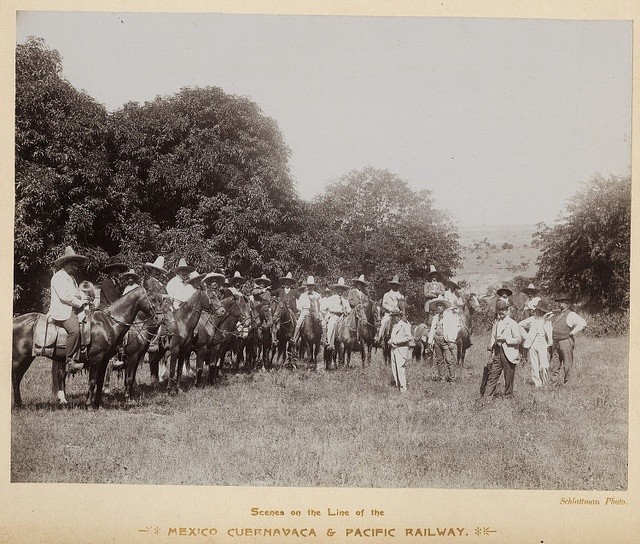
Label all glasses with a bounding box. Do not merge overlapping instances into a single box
[211,280,218,283]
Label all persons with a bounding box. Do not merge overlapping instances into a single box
[544,293,587,392]
[99,256,246,370]
[423,265,446,326]
[516,301,554,392]
[476,299,522,403]
[247,271,371,352]
[376,275,406,345]
[522,283,543,318]
[444,278,462,313]
[423,295,457,387]
[49,246,90,374]
[495,287,517,320]
[386,307,412,393]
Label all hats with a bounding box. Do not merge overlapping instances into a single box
[303,275,319,287]
[174,257,195,273]
[496,287,514,297]
[332,277,351,290]
[184,271,206,284]
[430,295,452,311]
[554,295,573,301]
[255,274,272,286]
[521,283,540,296]
[388,275,403,287]
[79,280,94,292]
[200,270,226,282]
[352,274,370,288]
[55,246,88,267]
[279,272,296,285]
[324,287,333,293]
[390,309,403,317]
[426,265,440,277]
[533,300,551,314]
[146,256,169,275]
[229,271,247,285]
[447,278,461,291]
[122,269,140,282]
[100,257,127,273]
[495,301,512,310]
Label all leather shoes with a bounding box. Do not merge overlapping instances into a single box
[65,361,85,374]
[107,362,124,371]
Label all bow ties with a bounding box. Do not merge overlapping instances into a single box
[286,286,288,288]
[308,291,313,294]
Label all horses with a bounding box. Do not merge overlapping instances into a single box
[343,298,382,368]
[274,296,301,367]
[150,292,251,389]
[378,313,431,361]
[256,320,276,369]
[124,280,225,391]
[302,296,323,371]
[413,310,472,365]
[54,282,177,406]
[324,300,370,369]
[368,298,422,369]
[178,320,248,378]
[229,293,274,367]
[12,275,165,411]
[185,299,261,380]
